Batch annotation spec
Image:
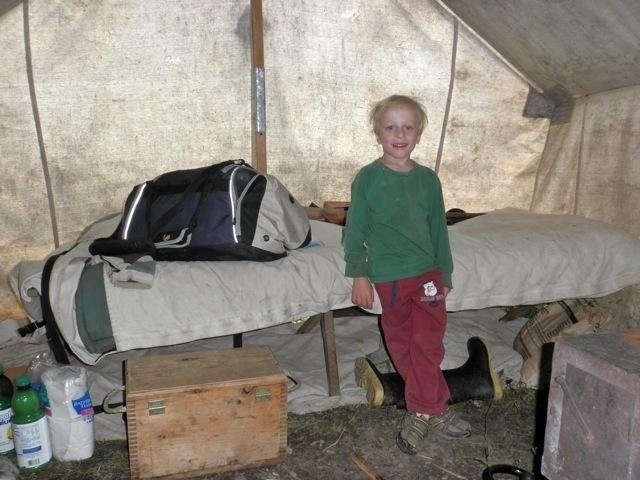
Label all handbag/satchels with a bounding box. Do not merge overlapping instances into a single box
[89,159,311,261]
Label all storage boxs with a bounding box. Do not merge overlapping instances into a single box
[102,344,291,480]
[540,327,640,479]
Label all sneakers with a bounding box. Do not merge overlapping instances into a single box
[397,411,429,455]
[429,407,471,438]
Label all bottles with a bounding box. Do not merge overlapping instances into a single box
[0,362,19,460]
[11,376,53,475]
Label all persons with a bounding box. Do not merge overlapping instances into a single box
[338,94,477,454]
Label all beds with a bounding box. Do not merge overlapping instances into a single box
[39,194,640,400]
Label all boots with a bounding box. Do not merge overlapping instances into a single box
[442,336,502,404]
[355,357,405,408]
